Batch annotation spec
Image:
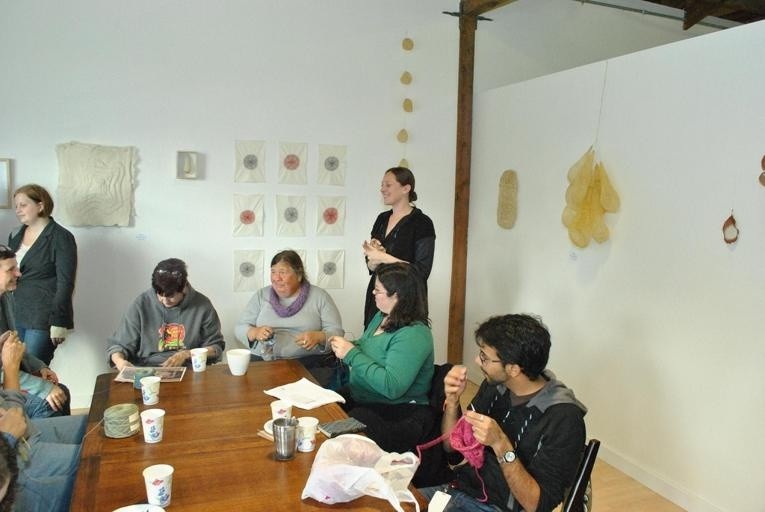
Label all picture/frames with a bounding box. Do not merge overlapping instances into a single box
[0,157,14,210]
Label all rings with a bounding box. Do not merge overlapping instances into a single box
[302,339,307,344]
[262,329,266,334]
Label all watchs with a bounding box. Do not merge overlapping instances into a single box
[497,448,520,464]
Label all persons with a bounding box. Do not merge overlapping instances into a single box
[0,403,77,512]
[326,261,435,452]
[104,258,225,371]
[0,245,71,418]
[233,248,344,362]
[0,183,79,368]
[0,327,92,481]
[415,314,588,512]
[362,166,437,334]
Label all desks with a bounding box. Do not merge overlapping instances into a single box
[69,360,429,512]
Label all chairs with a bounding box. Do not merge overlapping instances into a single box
[400,362,466,487]
[561,438,601,512]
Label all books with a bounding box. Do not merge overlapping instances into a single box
[263,377,346,411]
[113,366,187,383]
[318,416,368,441]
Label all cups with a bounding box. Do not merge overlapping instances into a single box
[140,408,166,444]
[226,348,253,376]
[142,463,176,508]
[272,418,301,462]
[270,399,293,420]
[190,347,209,373]
[139,376,163,406]
[297,416,321,453]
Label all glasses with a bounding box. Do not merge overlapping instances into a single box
[0,245,11,253]
[155,268,183,278]
[479,352,501,366]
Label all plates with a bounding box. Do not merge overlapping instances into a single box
[264,420,273,435]
